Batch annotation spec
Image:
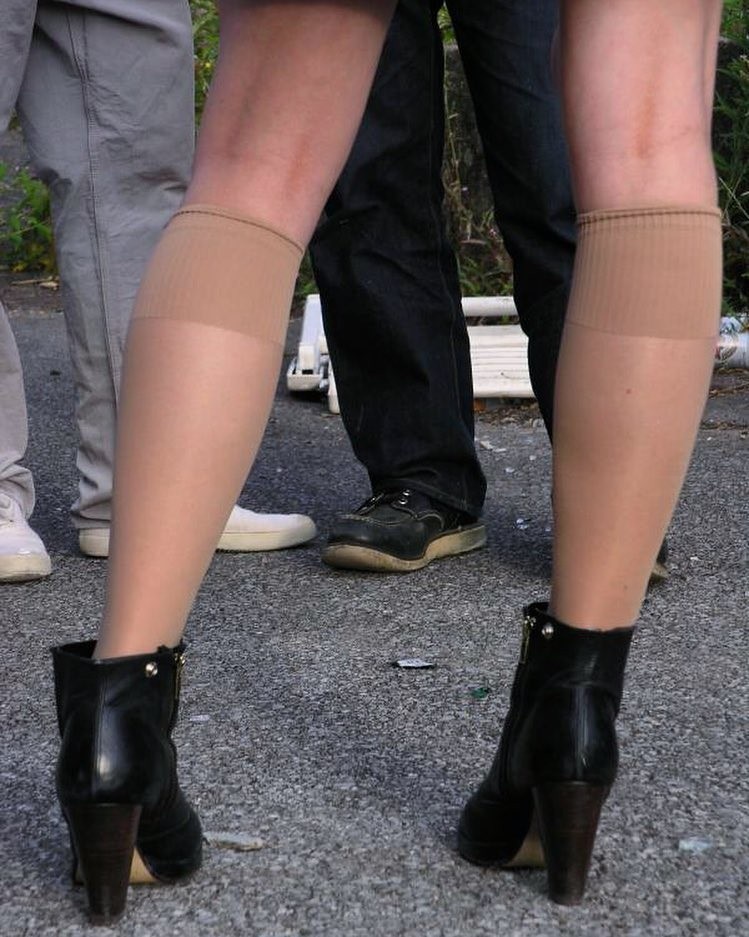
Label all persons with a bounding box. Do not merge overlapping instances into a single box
[0,0,318,583]
[52,0,722,926]
[307,0,673,583]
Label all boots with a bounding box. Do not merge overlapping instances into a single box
[47,638,204,923]
[458,601,636,905]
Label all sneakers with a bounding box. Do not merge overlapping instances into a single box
[78,504,317,556]
[0,493,52,581]
[318,488,488,573]
[652,540,670,579]
